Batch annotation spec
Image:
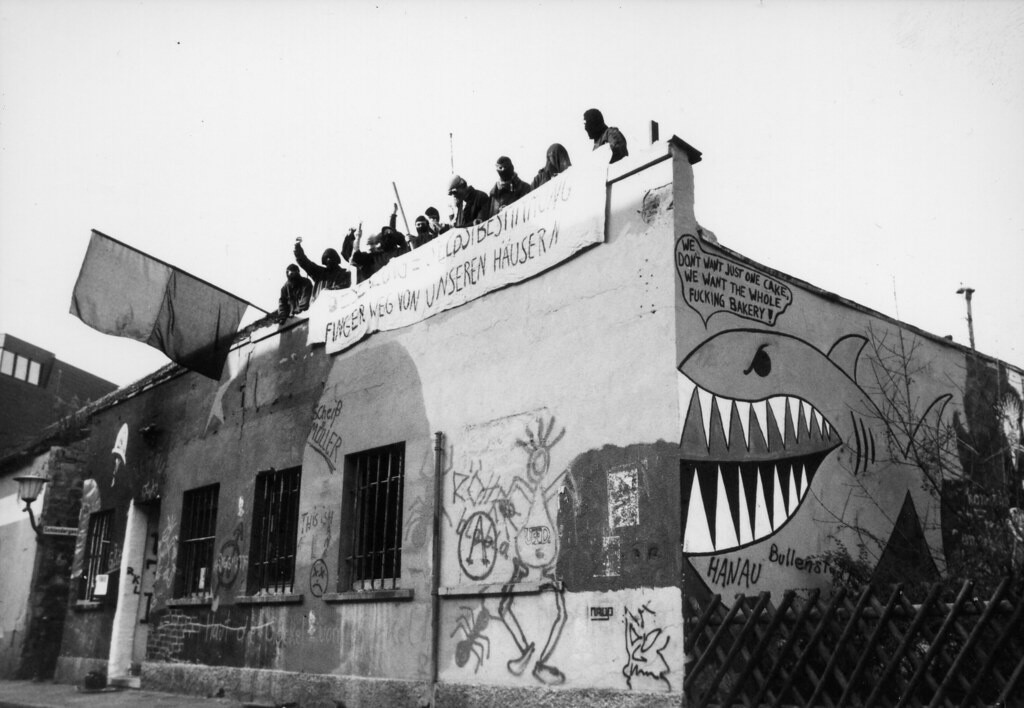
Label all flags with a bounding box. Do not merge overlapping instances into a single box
[69,232,248,382]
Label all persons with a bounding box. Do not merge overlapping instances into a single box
[473,156,530,225]
[342,225,410,284]
[405,207,451,249]
[279,264,313,324]
[294,242,351,305]
[531,144,571,192]
[583,109,628,164]
[448,176,489,227]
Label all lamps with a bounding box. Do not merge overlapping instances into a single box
[13,475,75,563]
